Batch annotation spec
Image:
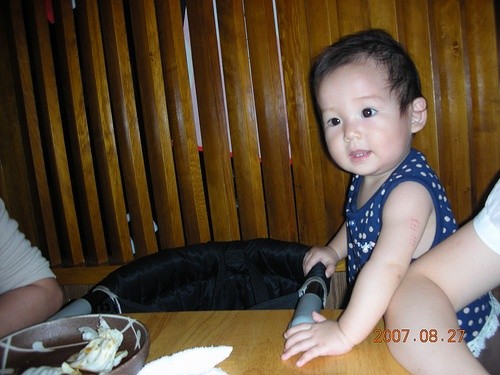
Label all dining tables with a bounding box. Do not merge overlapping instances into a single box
[89,308,414,375]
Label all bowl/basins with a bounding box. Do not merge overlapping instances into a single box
[0,314,150,375]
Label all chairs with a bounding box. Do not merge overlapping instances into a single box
[46,237,333,331]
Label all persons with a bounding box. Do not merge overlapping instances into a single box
[0,197,62,340]
[276,28,499,375]
[386,173,499,375]
